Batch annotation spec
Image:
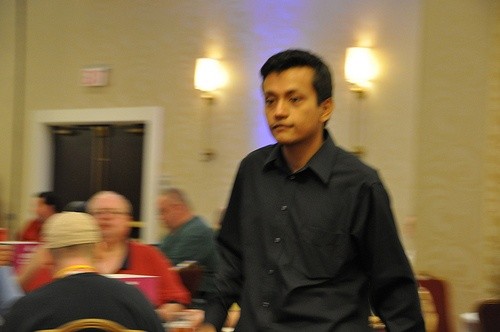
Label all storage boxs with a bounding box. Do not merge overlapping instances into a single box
[101,273,162,307]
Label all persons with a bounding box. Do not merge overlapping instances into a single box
[11,192,63,275]
[190,49,425,332]
[0,211,166,332]
[0,243,26,317]
[20,191,190,322]
[158,189,224,332]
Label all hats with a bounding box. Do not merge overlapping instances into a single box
[41,210,103,248]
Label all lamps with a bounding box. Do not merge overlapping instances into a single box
[193,57,222,101]
[344,46,377,94]
